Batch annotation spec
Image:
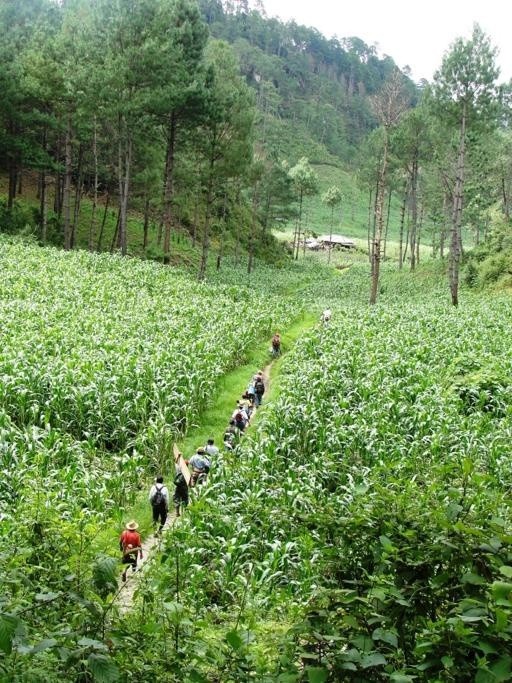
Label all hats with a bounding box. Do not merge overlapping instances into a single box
[207,439,214,443]
[126,520,139,531]
[198,447,205,453]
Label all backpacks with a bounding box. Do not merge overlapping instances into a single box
[257,382,263,394]
[236,409,244,421]
[152,485,167,508]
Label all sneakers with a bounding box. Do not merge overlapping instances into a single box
[122,572,126,581]
[154,530,162,537]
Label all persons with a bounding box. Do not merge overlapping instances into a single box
[272,331,282,359]
[149,475,169,539]
[173,370,265,519]
[321,305,333,327]
[119,520,143,581]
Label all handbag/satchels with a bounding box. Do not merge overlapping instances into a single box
[173,472,188,491]
